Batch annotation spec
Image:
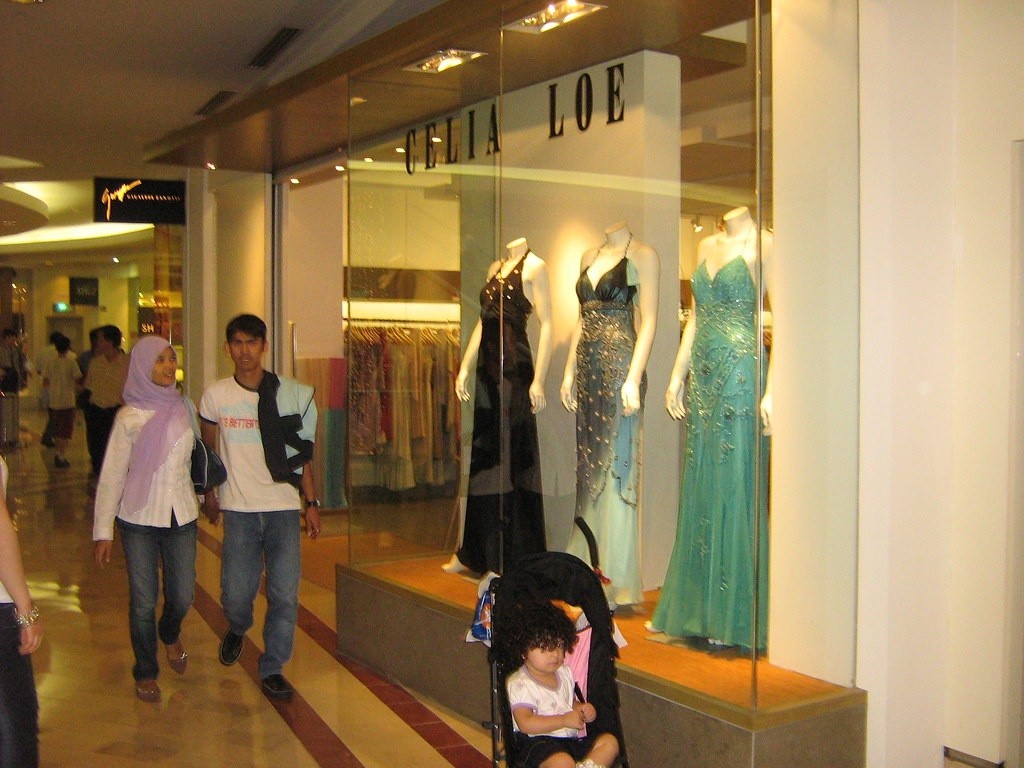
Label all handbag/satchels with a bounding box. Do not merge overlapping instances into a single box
[466,571,501,648]
[182,395,227,492]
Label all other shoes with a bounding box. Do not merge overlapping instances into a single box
[263,674,295,695]
[41,434,56,449]
[134,678,161,702]
[53,454,70,467]
[165,640,188,675]
[220,627,244,666]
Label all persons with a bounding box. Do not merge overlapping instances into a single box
[440,238,554,574]
[92,335,217,702]
[505,602,619,768]
[561,221,661,615]
[0,454,44,768]
[642,207,774,651]
[199,314,318,695]
[0,324,129,501]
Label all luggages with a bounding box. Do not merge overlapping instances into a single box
[0,391,19,448]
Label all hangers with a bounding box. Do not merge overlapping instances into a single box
[344,317,462,352]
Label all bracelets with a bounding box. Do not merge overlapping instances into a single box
[15,602,41,627]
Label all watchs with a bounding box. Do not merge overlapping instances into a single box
[306,499,320,508]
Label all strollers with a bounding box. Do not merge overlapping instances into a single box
[480,515,628,768]
